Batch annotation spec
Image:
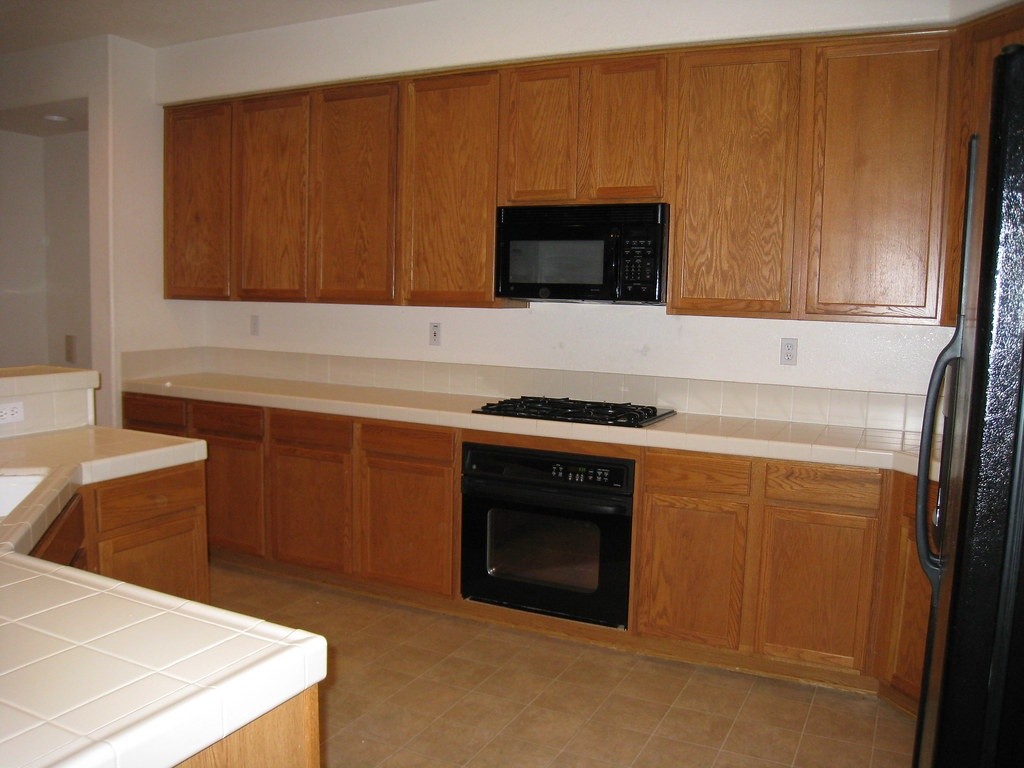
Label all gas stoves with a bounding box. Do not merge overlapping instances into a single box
[471,395,677,429]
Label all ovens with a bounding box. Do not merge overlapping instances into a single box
[460,442,636,630]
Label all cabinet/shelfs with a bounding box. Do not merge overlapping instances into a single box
[631,450,889,685]
[164,90,309,298]
[505,55,668,200]
[88,460,209,604]
[123,392,261,559]
[680,37,949,316]
[876,475,957,712]
[310,77,498,304]
[941,11,1024,325]
[266,406,458,607]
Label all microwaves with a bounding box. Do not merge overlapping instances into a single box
[496,203,668,307]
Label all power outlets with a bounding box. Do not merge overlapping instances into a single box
[250,316,259,337]
[64,334,79,364]
[780,338,798,366]
[429,323,442,347]
